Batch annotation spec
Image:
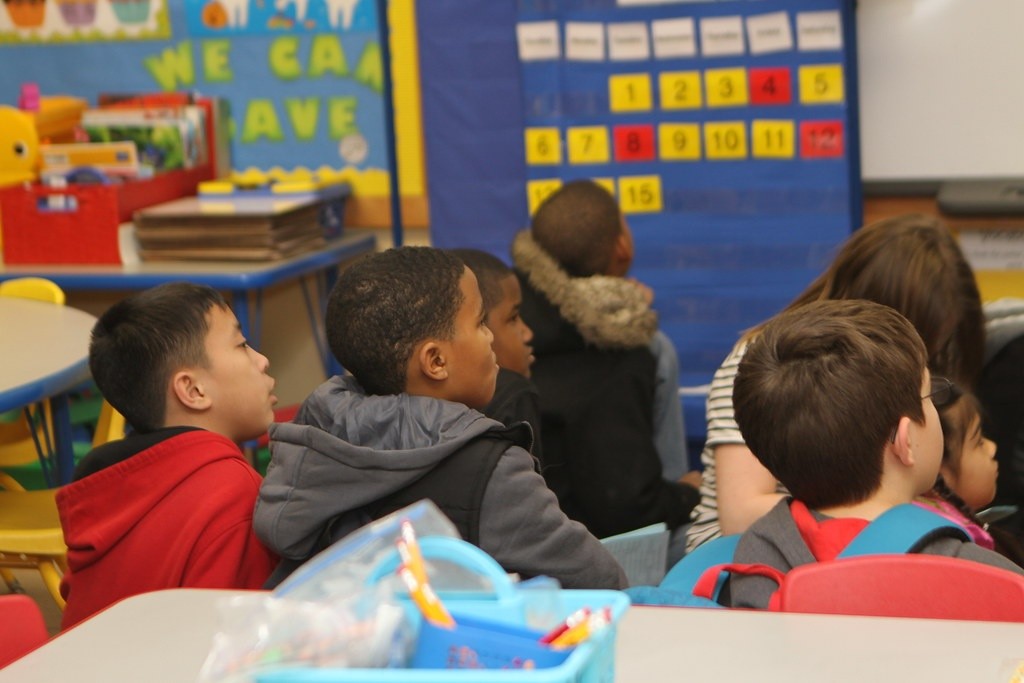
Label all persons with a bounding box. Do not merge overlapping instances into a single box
[457,249,541,469]
[58,280,279,635]
[513,181,703,538]
[666,299,1023,611]
[920,375,1023,571]
[257,243,630,591]
[687,214,995,555]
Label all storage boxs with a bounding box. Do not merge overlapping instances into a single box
[246,536,632,683]
[0,166,215,267]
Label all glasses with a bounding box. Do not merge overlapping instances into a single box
[891,377,955,446]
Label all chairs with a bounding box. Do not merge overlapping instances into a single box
[0,276,75,467]
[0,396,123,614]
[764,553,1023,623]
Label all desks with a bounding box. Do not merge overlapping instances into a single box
[0,295,103,487]
[3,583,1023,682]
[0,230,380,473]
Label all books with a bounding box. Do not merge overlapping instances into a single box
[32,86,349,265]
